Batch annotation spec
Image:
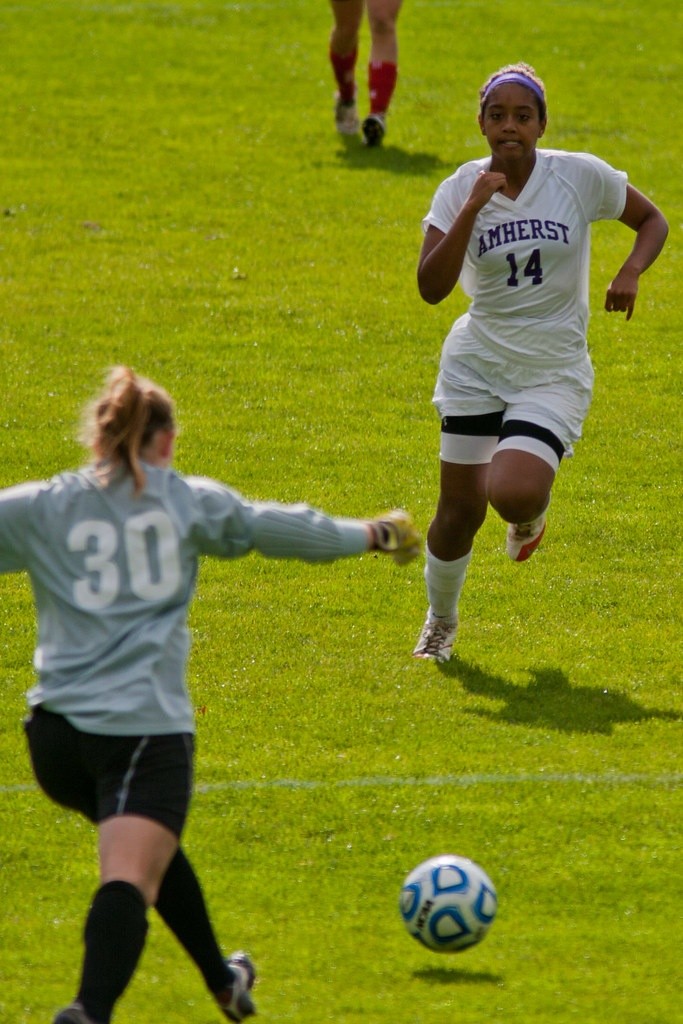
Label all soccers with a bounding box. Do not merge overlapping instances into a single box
[398,854,499,953]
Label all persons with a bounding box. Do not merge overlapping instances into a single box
[0,359,423,1024]
[414,61,669,662]
[325,0,404,149]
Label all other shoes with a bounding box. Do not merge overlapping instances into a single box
[361,113,386,146]
[336,102,358,135]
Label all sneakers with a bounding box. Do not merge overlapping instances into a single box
[54,1003,93,1024]
[507,517,545,561]
[220,951,256,1023]
[411,606,458,663]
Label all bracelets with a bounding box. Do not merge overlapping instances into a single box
[367,521,400,552]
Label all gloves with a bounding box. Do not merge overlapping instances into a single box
[367,510,423,566]
